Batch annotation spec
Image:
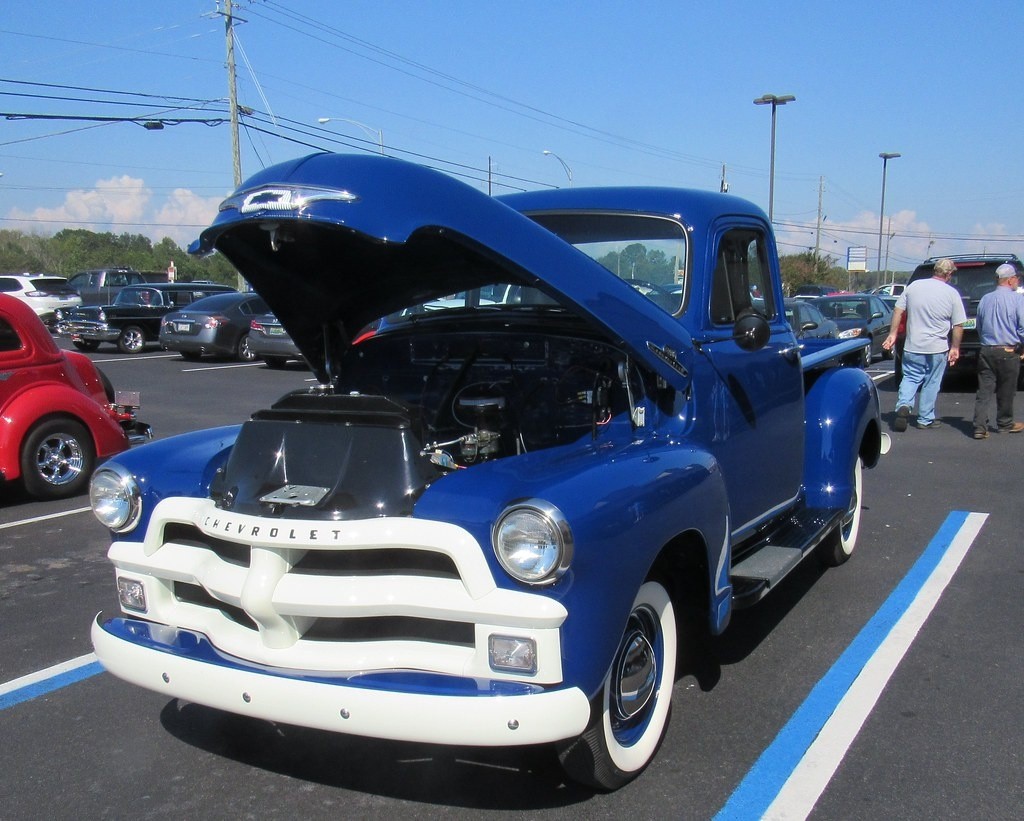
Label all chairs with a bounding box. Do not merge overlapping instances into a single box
[856,304,867,318]
[831,303,843,315]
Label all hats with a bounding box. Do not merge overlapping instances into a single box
[934,257,958,273]
[994,261,1024,279]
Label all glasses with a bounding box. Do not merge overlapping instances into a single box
[1012,276,1021,280]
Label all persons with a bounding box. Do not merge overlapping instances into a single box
[881,259,967,432]
[972,264,1024,438]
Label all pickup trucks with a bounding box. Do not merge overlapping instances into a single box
[88,154,893,792]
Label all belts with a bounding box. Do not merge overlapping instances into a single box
[991,346,1016,350]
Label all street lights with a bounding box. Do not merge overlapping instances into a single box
[317,118,384,157]
[541,149,574,188]
[876,153,902,296]
[752,94,795,228]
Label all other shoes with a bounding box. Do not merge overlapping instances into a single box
[974,428,991,439]
[893,406,909,432]
[917,420,941,429]
[998,422,1024,433]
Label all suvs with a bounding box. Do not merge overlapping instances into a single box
[68,267,150,307]
[114,270,169,286]
[0,274,82,330]
[895,254,1024,394]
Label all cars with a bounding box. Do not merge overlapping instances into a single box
[246,308,309,370]
[54,283,237,354]
[625,276,681,312]
[352,282,519,347]
[0,293,154,501]
[751,283,907,368]
[158,290,270,361]
